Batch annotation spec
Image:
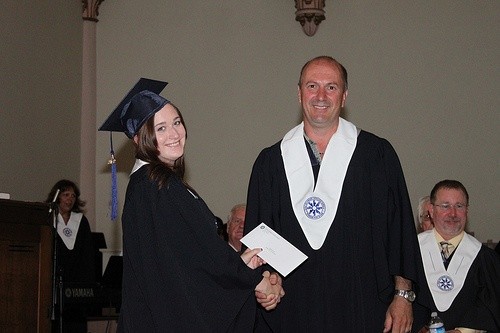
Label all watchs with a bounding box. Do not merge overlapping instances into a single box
[394,289,416,302]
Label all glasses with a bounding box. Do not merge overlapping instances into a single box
[421,214,430,220]
[434,202,468,210]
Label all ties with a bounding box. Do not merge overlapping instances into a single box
[440,243,449,261]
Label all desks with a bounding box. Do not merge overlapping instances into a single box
[0,198,52,333]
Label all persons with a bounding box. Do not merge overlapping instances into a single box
[226,202,246,254]
[99,77,285,333]
[45,179,98,333]
[241,56,432,333]
[417,179,500,333]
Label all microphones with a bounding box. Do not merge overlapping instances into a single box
[47,189,60,217]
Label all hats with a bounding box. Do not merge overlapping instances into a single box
[97,78,170,221]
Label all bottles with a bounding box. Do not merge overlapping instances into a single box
[429,312,445,333]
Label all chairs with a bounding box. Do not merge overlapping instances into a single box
[88,255,122,333]
